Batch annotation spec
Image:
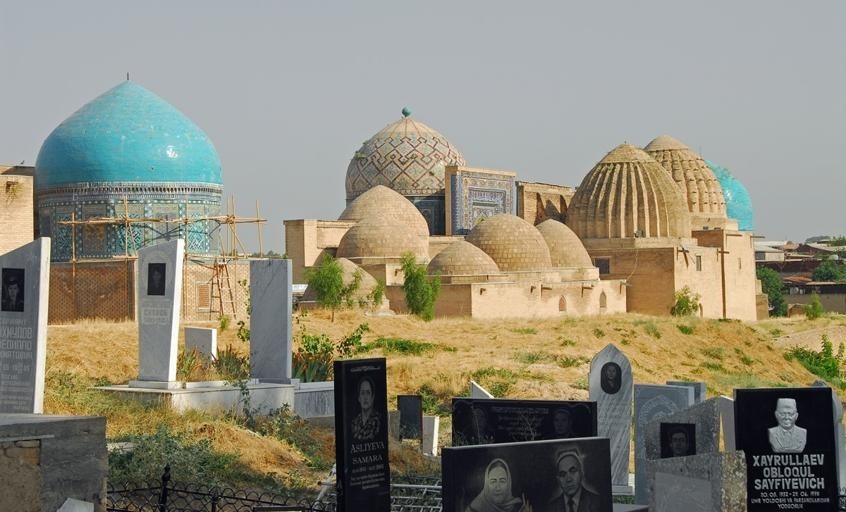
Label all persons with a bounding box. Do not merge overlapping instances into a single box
[0,270,24,310]
[664,426,694,458]
[550,410,575,438]
[147,262,165,296]
[602,362,623,394]
[544,449,611,511]
[766,396,808,454]
[346,378,383,442]
[461,457,538,512]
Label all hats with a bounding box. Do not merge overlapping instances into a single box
[551,441,586,473]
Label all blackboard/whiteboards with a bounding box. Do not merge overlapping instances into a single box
[397,395,423,440]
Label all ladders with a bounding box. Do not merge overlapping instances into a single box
[209,257,237,320]
